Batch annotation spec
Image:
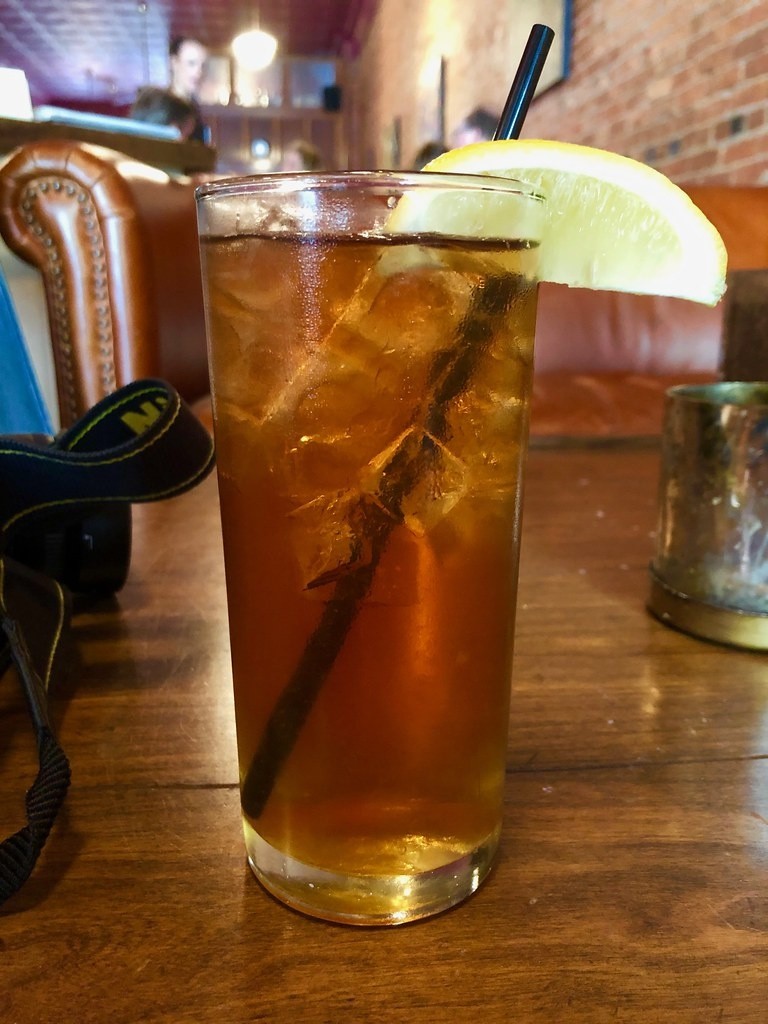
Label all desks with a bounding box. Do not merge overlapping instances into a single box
[0,430,768,1024]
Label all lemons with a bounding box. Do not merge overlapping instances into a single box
[375,140,729,307]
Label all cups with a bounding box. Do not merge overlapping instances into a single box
[644,381,768,654]
[190,173,544,927]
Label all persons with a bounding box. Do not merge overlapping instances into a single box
[130,35,208,145]
[282,140,323,173]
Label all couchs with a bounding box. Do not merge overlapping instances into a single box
[1,140,767,442]
